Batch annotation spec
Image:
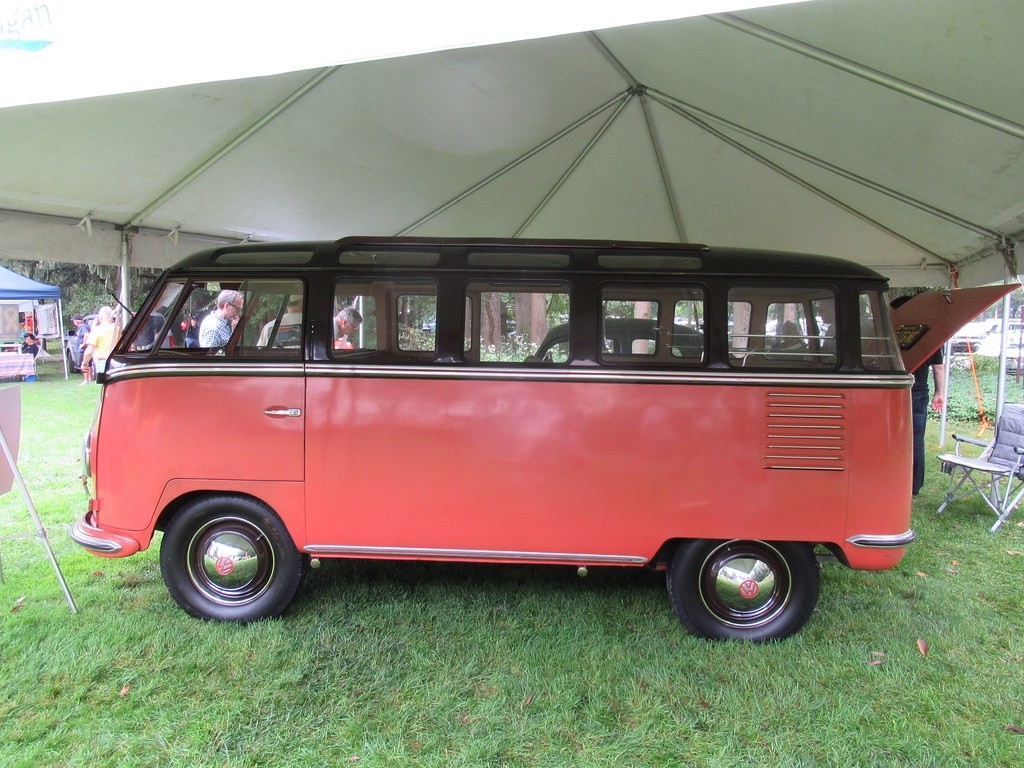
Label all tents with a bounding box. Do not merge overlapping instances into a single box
[0,265,68,380]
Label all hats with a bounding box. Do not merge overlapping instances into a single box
[70,315,83,321]
[24,334,35,340]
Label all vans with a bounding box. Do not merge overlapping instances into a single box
[66,314,176,380]
[64,236,914,644]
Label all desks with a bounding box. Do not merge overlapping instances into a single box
[0,344,21,347]
[0,353,34,381]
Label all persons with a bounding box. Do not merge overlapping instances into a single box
[4,333,38,357]
[257,295,304,347]
[888,295,945,494]
[70,315,93,386]
[121,316,155,351]
[200,290,244,347]
[191,316,198,329]
[334,308,363,341]
[81,306,120,372]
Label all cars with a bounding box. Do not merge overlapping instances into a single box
[1008,325,1024,347]
[949,324,988,354]
[525,315,739,373]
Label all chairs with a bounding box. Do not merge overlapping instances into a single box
[935,403,1024,532]
[18,344,42,382]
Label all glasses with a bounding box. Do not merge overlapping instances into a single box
[351,324,358,330]
[227,302,242,312]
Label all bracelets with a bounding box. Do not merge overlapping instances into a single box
[82,363,87,366]
[130,347,135,349]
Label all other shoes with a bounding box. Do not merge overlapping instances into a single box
[78,381,92,386]
